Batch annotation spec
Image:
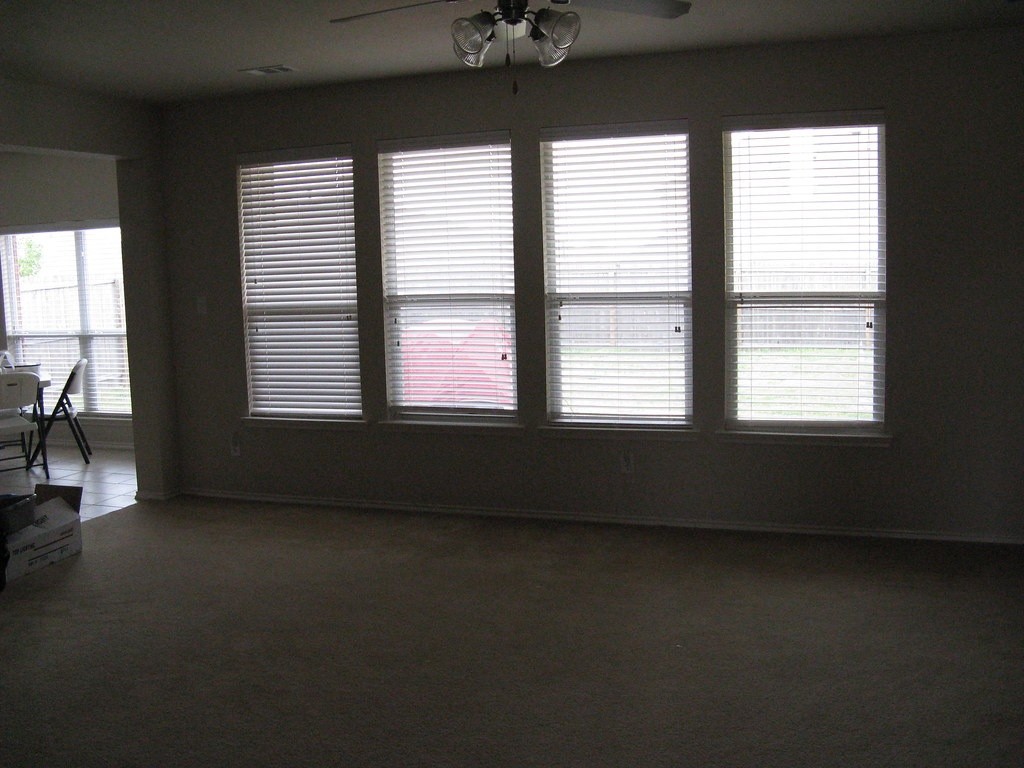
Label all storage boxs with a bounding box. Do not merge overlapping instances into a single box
[0,484,83,583]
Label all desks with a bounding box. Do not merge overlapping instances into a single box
[39,370,51,469]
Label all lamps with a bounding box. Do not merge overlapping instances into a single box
[452,0,581,95]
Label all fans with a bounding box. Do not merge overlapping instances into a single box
[329,0,691,23]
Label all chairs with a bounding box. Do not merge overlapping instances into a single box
[0,357,92,479]
